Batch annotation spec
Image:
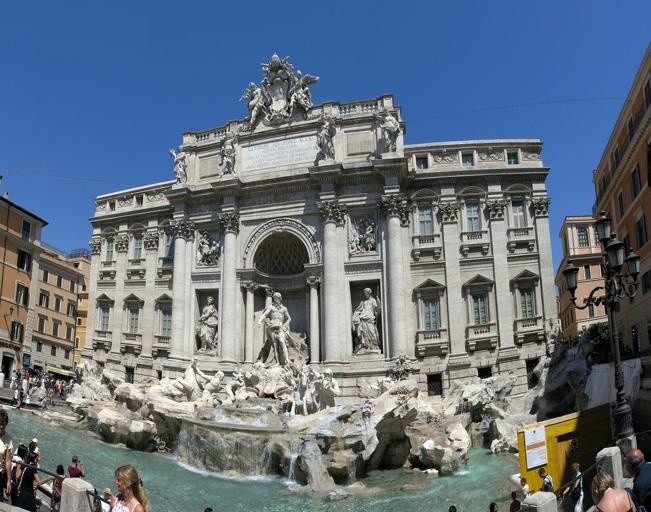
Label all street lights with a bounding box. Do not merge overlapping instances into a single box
[562,211,641,478]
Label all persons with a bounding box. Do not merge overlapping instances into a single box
[538,467,556,493]
[520,477,530,499]
[491,502,500,512]
[215,131,240,180]
[562,461,586,511]
[347,213,379,256]
[509,491,521,512]
[449,505,457,512]
[197,296,220,352]
[199,230,211,257]
[592,471,638,511]
[193,362,342,412]
[169,145,188,184]
[258,291,292,367]
[352,287,383,352]
[376,108,400,151]
[8,368,74,412]
[1,408,152,511]
[624,448,651,512]
[242,53,314,124]
[316,115,336,160]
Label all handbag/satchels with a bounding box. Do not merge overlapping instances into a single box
[571,486,580,500]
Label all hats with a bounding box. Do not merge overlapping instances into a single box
[68,466,81,476]
[103,488,112,494]
[31,438,38,442]
